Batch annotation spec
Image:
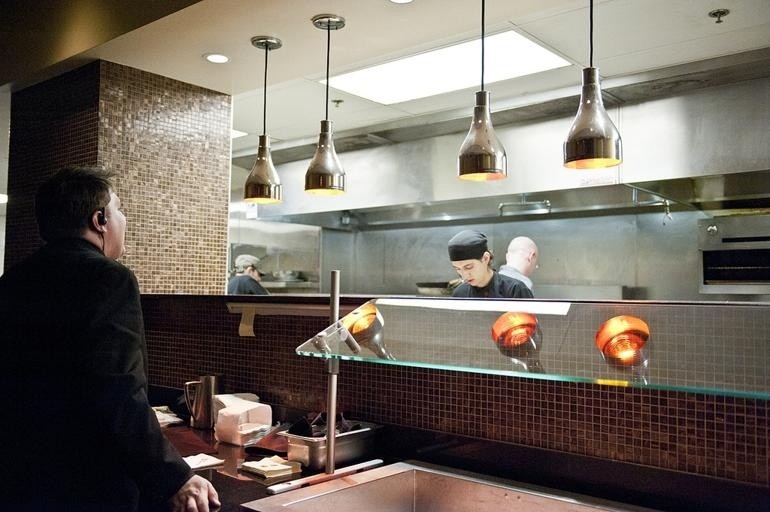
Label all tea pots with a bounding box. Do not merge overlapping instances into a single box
[183,372,222,431]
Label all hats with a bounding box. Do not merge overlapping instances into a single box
[234,253,260,268]
[447,228,494,262]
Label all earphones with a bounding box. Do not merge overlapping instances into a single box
[99,205,107,226]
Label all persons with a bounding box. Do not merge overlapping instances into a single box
[498,236,538,296]
[448,229,533,298]
[1,164,222,512]
[228,255,268,295]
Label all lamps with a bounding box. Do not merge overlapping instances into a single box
[562,1,623,171]
[345,304,397,360]
[244,36,281,203]
[595,315,651,384]
[491,311,544,373]
[456,0,507,182]
[304,13,345,195]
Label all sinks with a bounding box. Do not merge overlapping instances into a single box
[239,456,654,512]
